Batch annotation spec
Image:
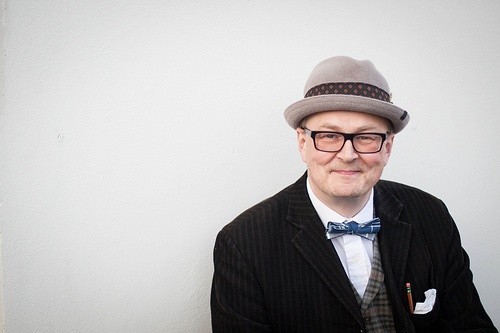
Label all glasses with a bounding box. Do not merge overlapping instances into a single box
[300,126,391,154]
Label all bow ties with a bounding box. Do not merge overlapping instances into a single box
[325,217,381,242]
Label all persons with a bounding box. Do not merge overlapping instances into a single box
[209,53,498,333]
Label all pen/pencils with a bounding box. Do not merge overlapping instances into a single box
[406,283,414,313]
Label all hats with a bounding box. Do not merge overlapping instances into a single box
[284,56,411,134]
